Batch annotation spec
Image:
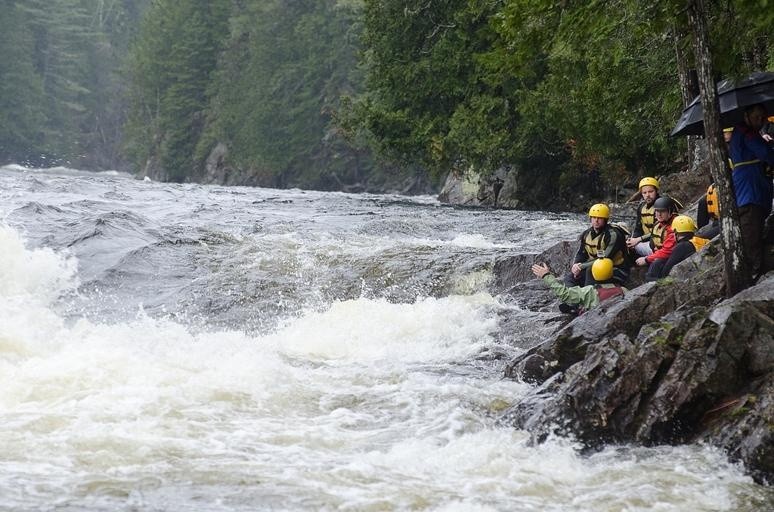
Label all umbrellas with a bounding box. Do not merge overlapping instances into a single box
[670,69,774,137]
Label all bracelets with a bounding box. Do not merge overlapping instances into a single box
[641,236,643,241]
[541,272,550,279]
[644,257,648,263]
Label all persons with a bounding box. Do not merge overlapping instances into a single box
[556,204,630,313]
[722,116,773,171]
[645,214,697,281]
[529,252,630,316]
[726,102,774,284]
[625,177,661,257]
[634,197,677,268]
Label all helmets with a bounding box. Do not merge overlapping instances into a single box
[654,197,674,216]
[591,258,614,282]
[589,203,610,220]
[672,215,697,233]
[639,177,660,192]
